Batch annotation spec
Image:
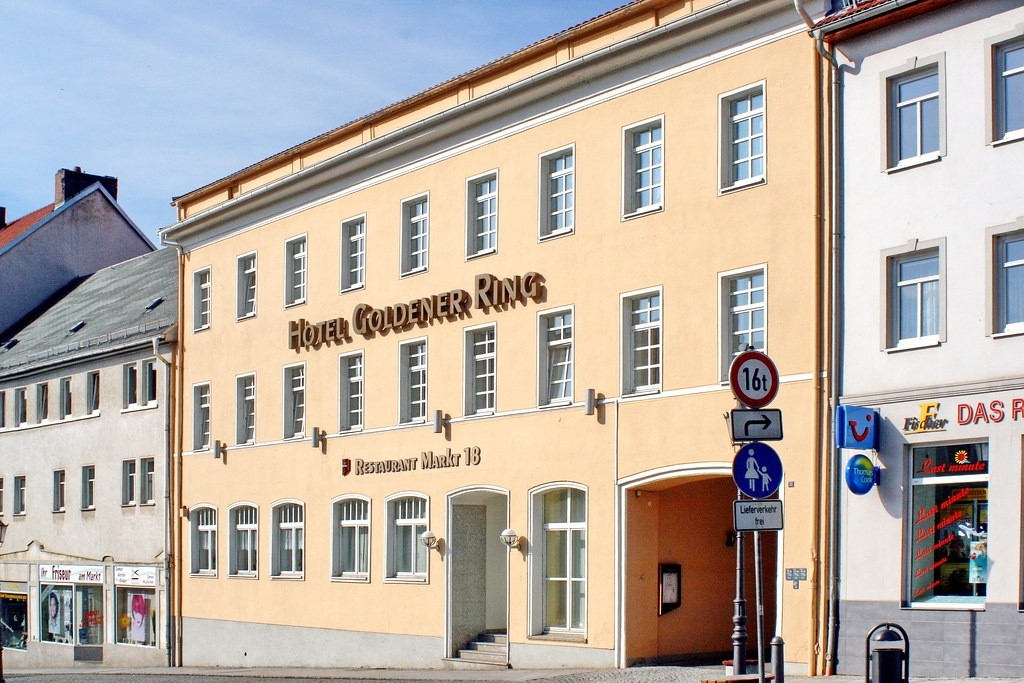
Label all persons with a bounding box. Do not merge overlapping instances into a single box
[11,616,20,646]
[49,592,60,633]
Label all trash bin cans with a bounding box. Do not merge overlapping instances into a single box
[864,621,911,683]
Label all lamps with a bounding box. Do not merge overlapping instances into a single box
[433,410,441,434]
[584,388,595,416]
[311,426,320,447]
[213,439,221,459]
[419,531,439,552]
[500,528,520,553]
